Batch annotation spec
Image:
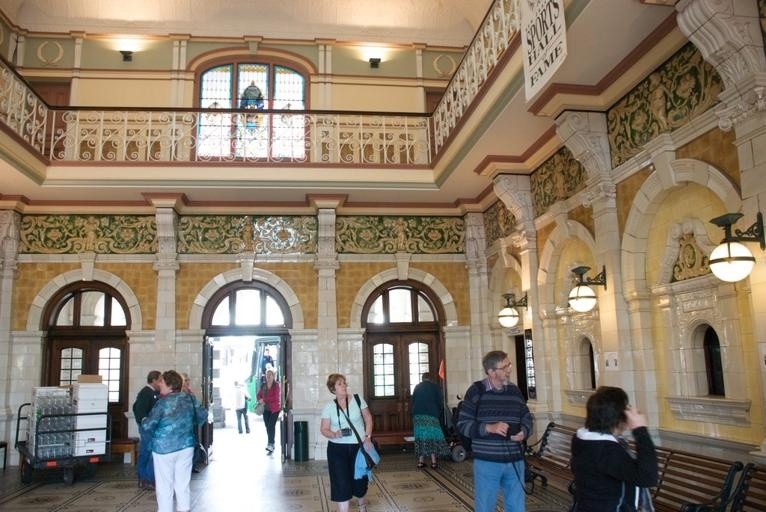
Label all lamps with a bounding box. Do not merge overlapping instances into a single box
[498,293,528,327]
[569,267,605,312]
[707,213,764,281]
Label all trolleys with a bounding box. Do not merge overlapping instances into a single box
[437,407,472,461]
[14,403,111,485]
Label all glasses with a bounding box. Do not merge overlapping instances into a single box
[495,363,512,371]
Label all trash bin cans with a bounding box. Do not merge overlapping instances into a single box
[293,420,309,464]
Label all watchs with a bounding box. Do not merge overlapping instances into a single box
[365,435,372,439]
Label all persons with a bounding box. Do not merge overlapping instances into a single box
[320,373,373,512]
[410,371,447,469]
[262,348,274,375]
[181,374,192,395]
[131,371,161,491]
[232,380,252,434]
[256,371,282,452]
[455,349,534,512]
[569,386,624,512]
[141,369,208,512]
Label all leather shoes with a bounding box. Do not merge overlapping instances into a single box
[142,479,155,490]
[138,481,144,487]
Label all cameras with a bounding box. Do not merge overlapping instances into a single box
[339,427,353,438]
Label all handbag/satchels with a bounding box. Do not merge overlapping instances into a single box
[453,382,484,452]
[190,394,207,463]
[254,384,265,415]
[333,398,381,470]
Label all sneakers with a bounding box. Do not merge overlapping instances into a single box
[265,446,274,453]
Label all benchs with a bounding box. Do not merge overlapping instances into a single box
[112,436,139,466]
[525,422,743,512]
[696,464,766,512]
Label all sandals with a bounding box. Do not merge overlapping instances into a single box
[431,463,439,470]
[357,503,367,512]
[417,462,426,468]
